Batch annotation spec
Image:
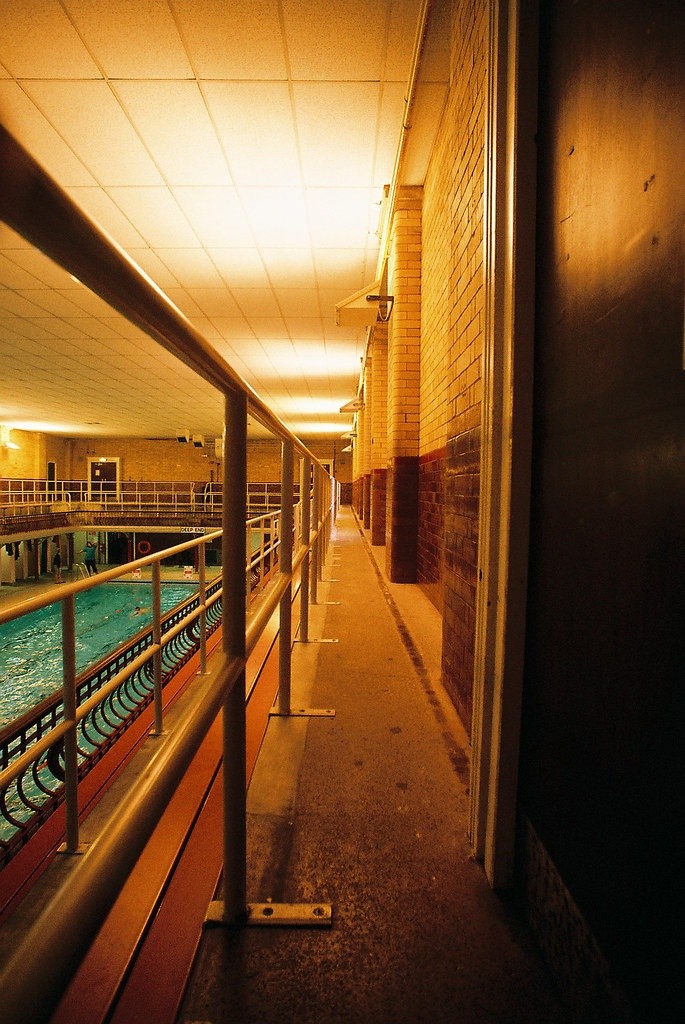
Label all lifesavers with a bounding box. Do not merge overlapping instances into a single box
[138,540,151,554]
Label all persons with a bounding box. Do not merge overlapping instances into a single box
[74,541,99,577]
[53,548,66,584]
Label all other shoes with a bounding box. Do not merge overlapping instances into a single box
[61,581,67,583]
[55,582,60,584]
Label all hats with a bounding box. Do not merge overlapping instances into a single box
[134,607,140,610]
[57,548,62,553]
